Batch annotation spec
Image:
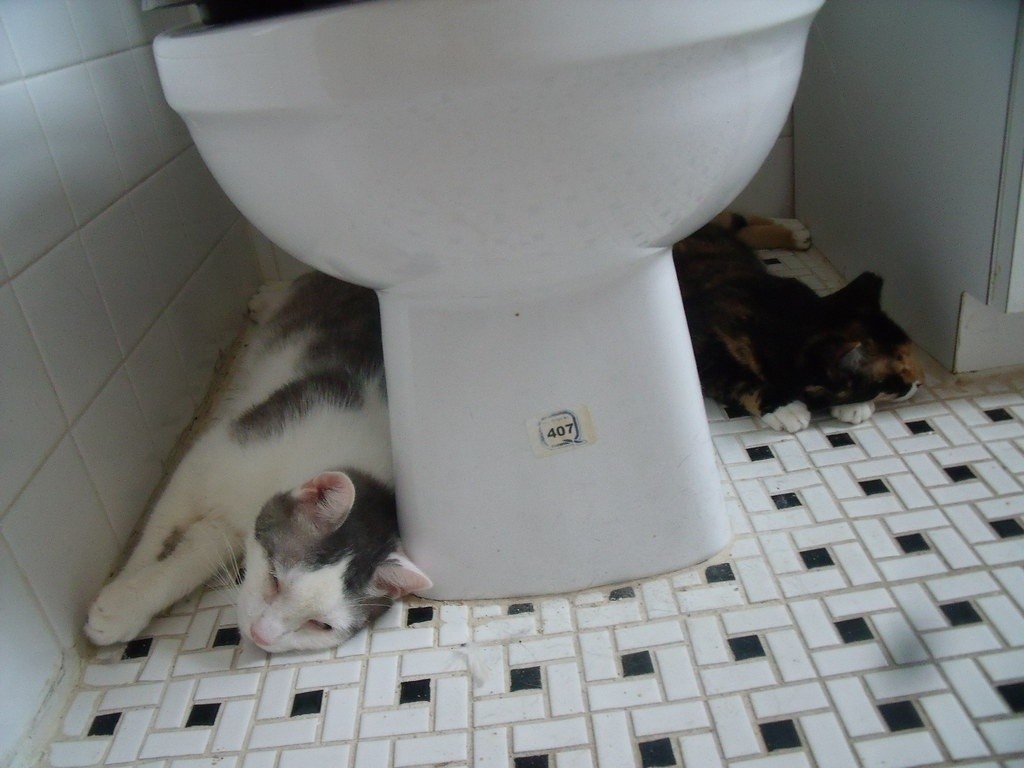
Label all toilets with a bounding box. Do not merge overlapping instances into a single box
[148,0,845,598]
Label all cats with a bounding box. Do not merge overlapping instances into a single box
[80,268,434,655]
[672,204,926,433]
